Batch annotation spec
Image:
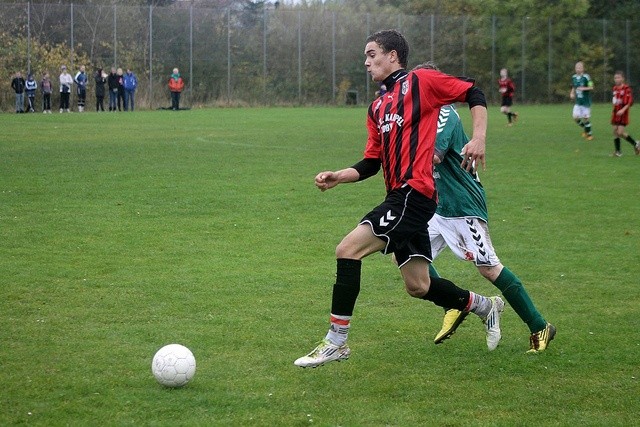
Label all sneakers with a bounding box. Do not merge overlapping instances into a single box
[294,338,350,368]
[481,296,505,351]
[527,322,556,355]
[433,309,470,344]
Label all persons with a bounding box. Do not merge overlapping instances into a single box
[292,27,503,370]
[11,64,137,112]
[167,68,183,109]
[570,61,595,140]
[498,67,519,128]
[611,70,639,157]
[408,61,556,354]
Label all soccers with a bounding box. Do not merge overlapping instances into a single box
[151,343,197,388]
